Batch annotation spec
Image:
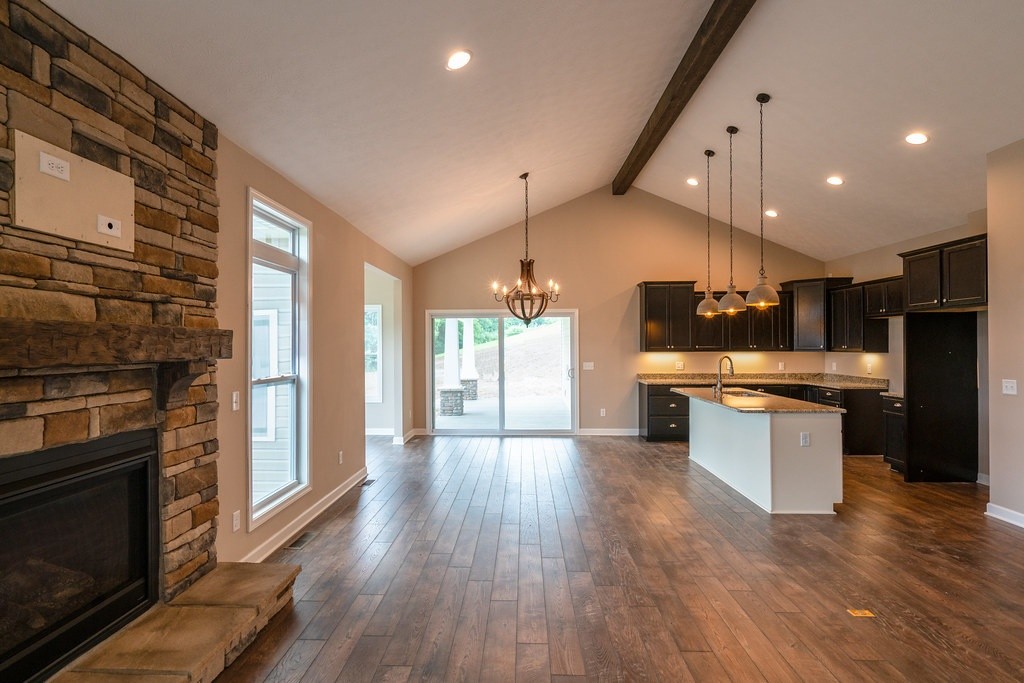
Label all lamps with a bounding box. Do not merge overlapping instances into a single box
[717,124,746,317]
[745,92,780,311]
[696,148,722,320]
[491,171,560,328]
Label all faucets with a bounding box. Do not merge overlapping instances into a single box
[712,355,734,399]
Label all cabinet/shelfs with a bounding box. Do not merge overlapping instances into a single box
[896,232,989,313]
[636,281,697,352]
[637,381,805,442]
[778,276,854,351]
[692,290,725,351]
[879,391,906,474]
[862,274,904,320]
[828,283,889,353]
[806,385,888,455]
[727,290,772,351]
[772,290,795,351]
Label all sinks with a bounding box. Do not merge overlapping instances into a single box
[721,391,772,397]
